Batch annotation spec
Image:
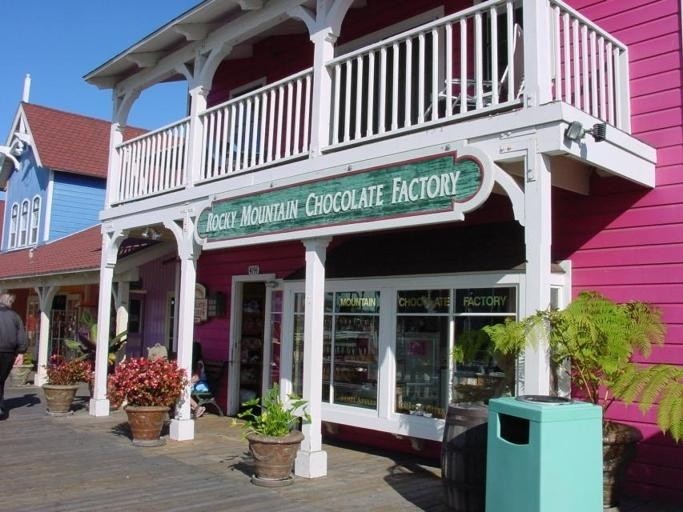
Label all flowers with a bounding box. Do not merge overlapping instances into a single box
[38,353,91,386]
[106,358,186,407]
[13,353,34,366]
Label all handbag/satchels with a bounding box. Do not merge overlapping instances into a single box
[193,380,209,393]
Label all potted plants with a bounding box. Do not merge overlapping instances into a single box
[449,288,682,512]
[229,383,312,481]
[65,311,127,412]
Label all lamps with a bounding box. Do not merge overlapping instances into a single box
[565,120,607,142]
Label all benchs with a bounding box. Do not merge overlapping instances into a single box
[189,360,225,418]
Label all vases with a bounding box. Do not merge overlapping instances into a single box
[8,366,33,385]
[42,385,76,413]
[122,407,171,440]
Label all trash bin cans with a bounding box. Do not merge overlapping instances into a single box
[485,395,604,512]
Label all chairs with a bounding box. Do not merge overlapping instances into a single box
[422,25,526,122]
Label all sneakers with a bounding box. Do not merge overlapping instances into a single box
[195,406,206,418]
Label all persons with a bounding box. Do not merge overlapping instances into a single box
[186,342,212,419]
[0,293,29,420]
